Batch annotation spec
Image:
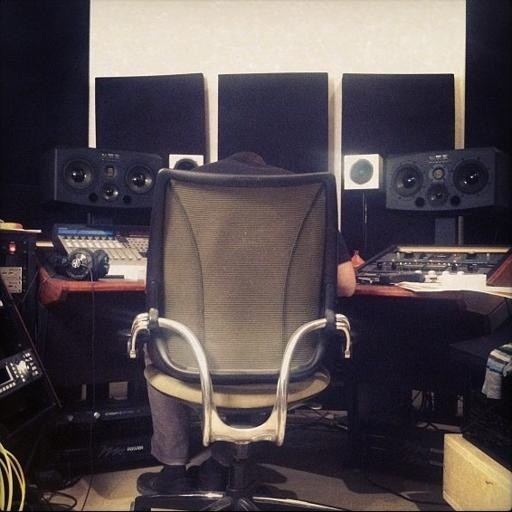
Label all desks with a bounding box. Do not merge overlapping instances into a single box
[38,240,511,337]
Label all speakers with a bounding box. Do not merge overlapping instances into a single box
[385,146,511,212]
[39,149,164,210]
[168,154,204,171]
[343,154,383,190]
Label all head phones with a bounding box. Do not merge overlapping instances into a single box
[47,247,110,281]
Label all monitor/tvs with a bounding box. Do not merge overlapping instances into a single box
[0,274,64,451]
